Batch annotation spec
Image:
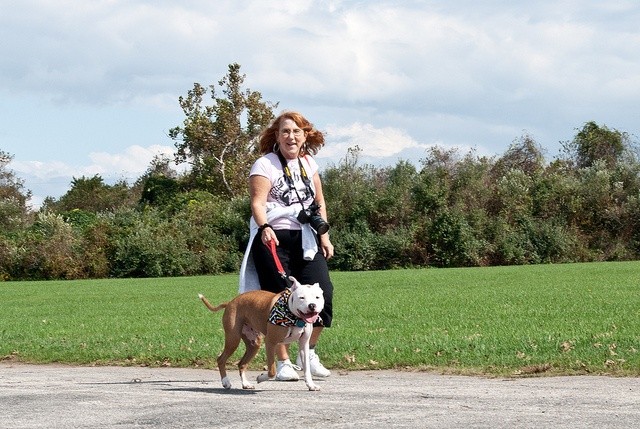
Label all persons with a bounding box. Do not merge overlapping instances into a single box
[241,111,335,381]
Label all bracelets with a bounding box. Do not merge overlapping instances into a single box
[258,224,273,235]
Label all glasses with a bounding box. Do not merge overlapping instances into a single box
[279,128,305,135]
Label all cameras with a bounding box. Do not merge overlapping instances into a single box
[297,204,330,236]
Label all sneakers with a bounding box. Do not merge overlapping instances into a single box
[297,349,331,377]
[276,360,301,381]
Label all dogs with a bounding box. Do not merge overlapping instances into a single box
[198,276,325,391]
